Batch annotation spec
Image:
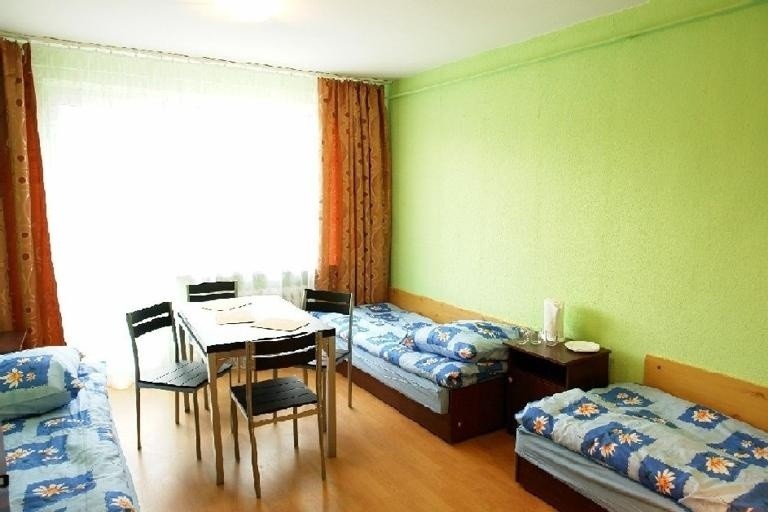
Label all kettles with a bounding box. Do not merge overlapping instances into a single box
[543,295,566,342]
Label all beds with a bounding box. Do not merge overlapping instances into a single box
[0,361,141,512]
[514,353,768,512]
[309,287,529,444]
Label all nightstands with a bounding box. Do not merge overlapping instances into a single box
[0,330,27,353]
[503,333,611,437]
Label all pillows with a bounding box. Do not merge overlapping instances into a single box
[0,346,87,422]
[399,320,527,363]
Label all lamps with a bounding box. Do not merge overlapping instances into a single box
[543,298,565,347]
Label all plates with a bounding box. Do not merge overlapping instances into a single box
[564,340,601,354]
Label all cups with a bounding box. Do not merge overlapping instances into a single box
[516,323,559,347]
